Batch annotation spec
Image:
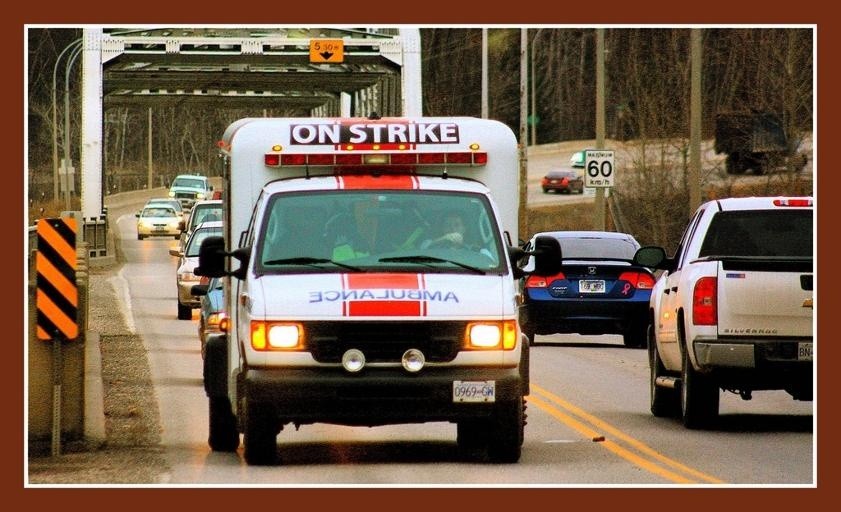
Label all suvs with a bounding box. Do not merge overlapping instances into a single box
[631,192,813,430]
[169,174,214,209]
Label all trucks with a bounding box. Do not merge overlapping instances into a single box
[224,113,532,462]
[714,110,809,177]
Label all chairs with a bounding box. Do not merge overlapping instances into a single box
[274,201,331,264]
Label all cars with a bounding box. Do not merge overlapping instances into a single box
[135,204,182,240]
[168,220,225,320]
[520,230,657,349]
[541,169,583,194]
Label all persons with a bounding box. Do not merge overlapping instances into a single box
[418,210,496,265]
